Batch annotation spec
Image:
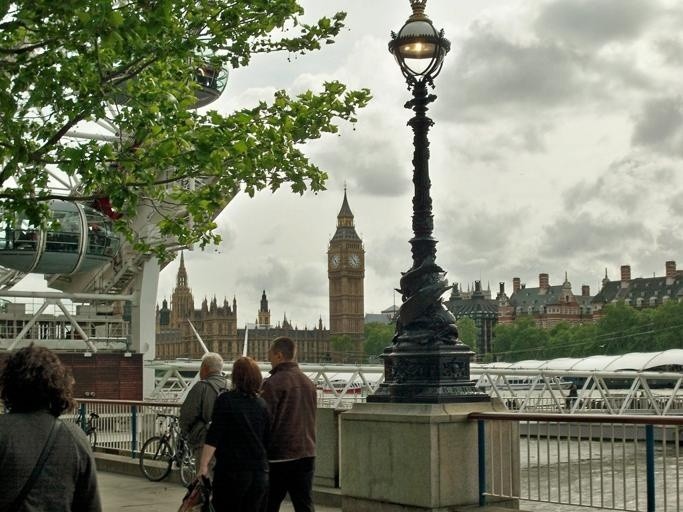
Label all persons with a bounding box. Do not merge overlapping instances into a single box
[178,352,232,479]
[568,384,578,408]
[0,346,104,512]
[197,355,272,512]
[262,336,318,512]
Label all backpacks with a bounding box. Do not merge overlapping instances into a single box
[197,378,232,431]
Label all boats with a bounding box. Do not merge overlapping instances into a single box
[470,348,683,441]
[307,372,374,396]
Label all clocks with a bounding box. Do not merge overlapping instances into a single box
[331,251,365,274]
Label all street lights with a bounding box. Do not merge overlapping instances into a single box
[372,1,484,393]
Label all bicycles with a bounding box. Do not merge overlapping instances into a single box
[73,405,100,451]
[140,413,214,485]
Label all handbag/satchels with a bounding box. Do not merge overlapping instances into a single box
[259,451,270,487]
[178,475,216,512]
